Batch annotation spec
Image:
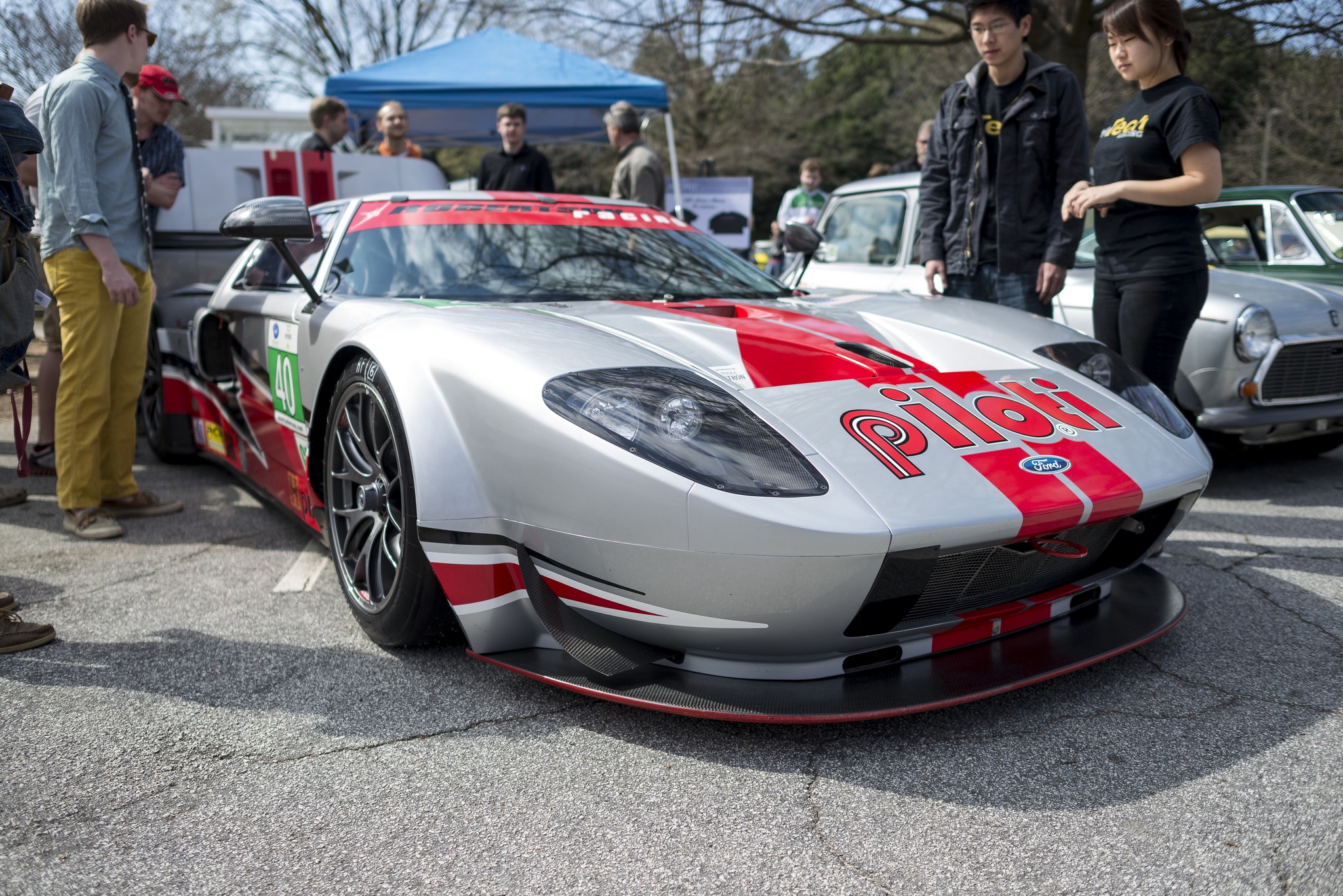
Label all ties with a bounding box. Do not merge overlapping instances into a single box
[120,81,154,271]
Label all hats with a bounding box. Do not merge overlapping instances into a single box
[138,64,189,106]
[602,100,638,127]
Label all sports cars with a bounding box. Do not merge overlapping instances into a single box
[140,188,1211,724]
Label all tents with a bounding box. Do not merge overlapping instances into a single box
[325,27,685,226]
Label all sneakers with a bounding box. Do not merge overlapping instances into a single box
[29,440,57,476]
[0,615,56,654]
[0,591,17,612]
[0,486,27,507]
[101,492,182,516]
[62,506,122,538]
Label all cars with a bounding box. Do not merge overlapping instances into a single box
[780,172,1343,456]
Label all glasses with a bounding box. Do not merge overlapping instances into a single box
[918,138,928,144]
[966,22,1015,36]
[137,25,157,46]
[381,114,404,122]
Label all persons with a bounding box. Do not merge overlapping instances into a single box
[221,264,268,371]
[366,101,430,158]
[22,48,140,470]
[1061,1,1224,558]
[129,63,189,228]
[603,99,664,214]
[917,0,1086,323]
[0,84,56,657]
[741,121,1343,279]
[477,104,557,193]
[302,97,349,153]
[39,0,184,539]
